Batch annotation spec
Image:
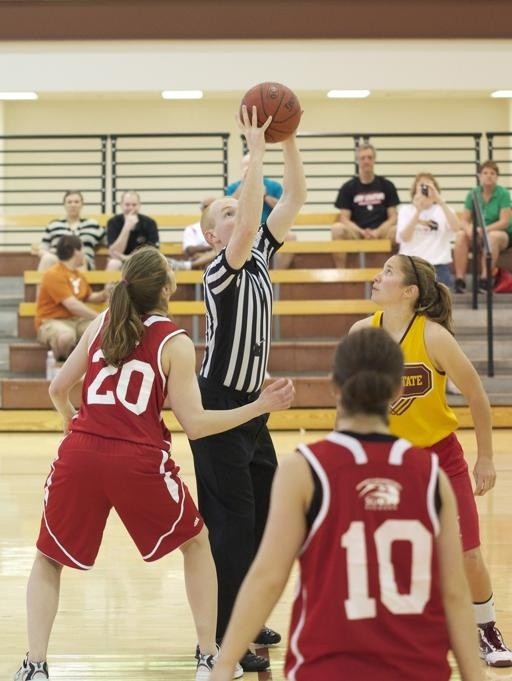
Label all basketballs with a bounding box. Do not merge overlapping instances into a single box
[240,83,301,143]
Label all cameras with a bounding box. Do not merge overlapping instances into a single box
[421,185,427,196]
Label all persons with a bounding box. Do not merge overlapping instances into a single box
[222,156,296,268]
[206,326,491,681]
[102,192,158,307]
[395,174,461,396]
[190,106,308,671]
[349,255,512,668]
[33,237,99,378]
[15,246,295,681]
[35,189,109,277]
[331,144,401,270]
[183,193,225,271]
[452,161,512,293]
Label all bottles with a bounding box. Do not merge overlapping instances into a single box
[46,351,55,382]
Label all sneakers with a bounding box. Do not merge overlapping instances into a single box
[249,626,282,650]
[14,651,48,680]
[477,621,512,666]
[195,644,244,681]
[194,645,270,671]
